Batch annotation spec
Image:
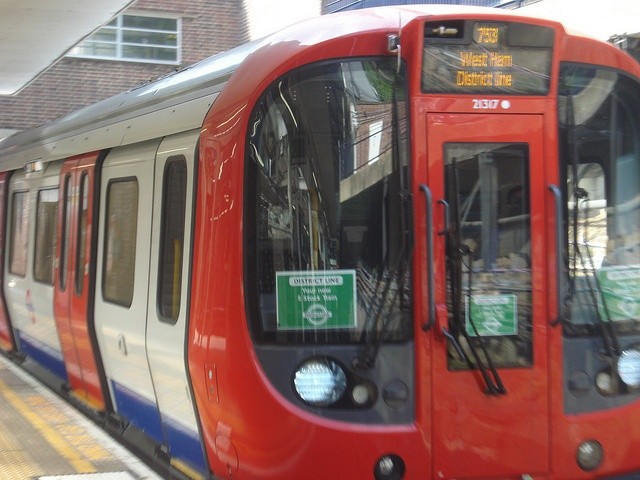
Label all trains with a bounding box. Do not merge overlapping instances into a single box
[0,4,640,480]
